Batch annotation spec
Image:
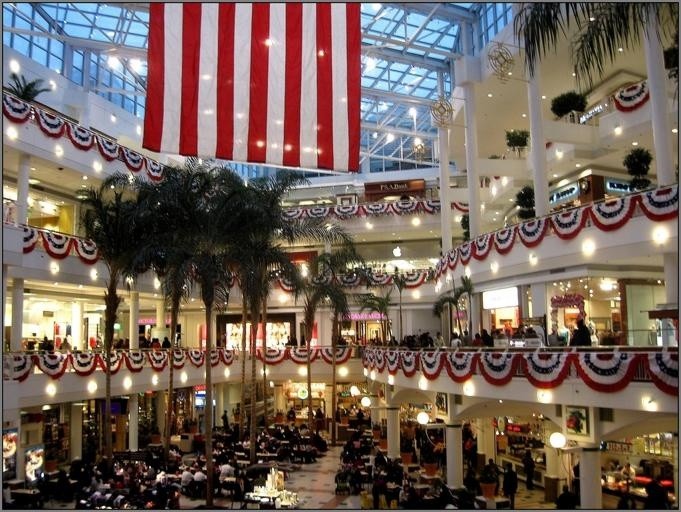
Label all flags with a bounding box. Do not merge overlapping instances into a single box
[137,1,362,175]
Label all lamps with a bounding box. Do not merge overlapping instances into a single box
[356,394,395,417]
[547,431,588,454]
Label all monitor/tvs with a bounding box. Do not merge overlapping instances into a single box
[195,396,206,408]
[102,397,129,415]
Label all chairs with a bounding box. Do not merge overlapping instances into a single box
[335,423,515,510]
[3,423,326,511]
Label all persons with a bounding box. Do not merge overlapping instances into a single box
[621,462,636,481]
[44,341,54,354]
[555,484,578,509]
[315,407,324,433]
[574,318,592,352]
[335,407,342,423]
[642,479,672,509]
[59,337,72,354]
[338,336,343,348]
[285,335,307,349]
[616,491,637,509]
[113,335,172,352]
[287,407,297,420]
[221,410,230,428]
[40,336,48,354]
[74,418,500,510]
[573,462,581,506]
[357,408,364,423]
[521,450,536,490]
[570,329,580,352]
[525,327,539,338]
[368,328,501,352]
[344,408,349,417]
[512,324,527,338]
[502,462,519,509]
